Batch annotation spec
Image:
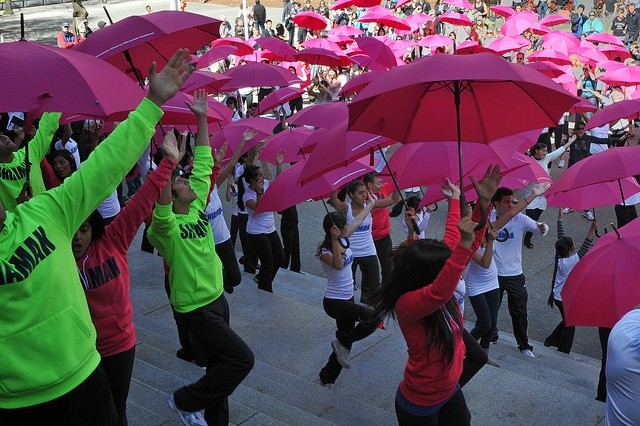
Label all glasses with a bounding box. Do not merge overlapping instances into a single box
[577,127,584,131]
[517,58,522,60]
[582,68,587,70]
[226,101,232,105]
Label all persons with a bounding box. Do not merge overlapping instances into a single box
[225,96,242,121]
[144,5,153,14]
[447,30,459,49]
[613,0,640,12]
[580,33,588,41]
[562,121,629,221]
[351,171,383,291]
[238,59,253,107]
[543,208,596,355]
[571,4,588,39]
[285,9,296,37]
[549,121,566,168]
[596,0,608,19]
[582,10,604,35]
[314,191,383,392]
[484,29,496,47]
[623,58,639,65]
[384,0,431,18]
[587,124,610,154]
[613,192,639,229]
[73,1,93,34]
[340,44,348,51]
[0,47,194,425]
[465,200,501,367]
[569,55,583,82]
[369,162,505,426]
[0,111,62,212]
[237,148,287,293]
[277,203,302,274]
[219,18,287,39]
[365,24,404,42]
[472,15,487,45]
[252,0,266,34]
[412,31,421,59]
[626,3,639,51]
[282,0,328,16]
[514,52,525,64]
[292,26,325,47]
[239,1,253,22]
[627,43,640,58]
[523,31,542,52]
[366,170,398,282]
[95,189,122,222]
[123,127,198,202]
[145,88,256,426]
[595,327,611,403]
[246,100,259,118]
[180,0,187,11]
[330,178,401,329]
[57,23,75,49]
[71,132,179,426]
[524,133,578,250]
[434,46,445,54]
[258,60,277,102]
[435,0,471,19]
[582,86,629,149]
[308,66,362,103]
[465,36,471,42]
[288,67,308,114]
[332,9,360,29]
[471,0,488,18]
[511,0,575,19]
[98,21,107,30]
[486,187,549,358]
[605,307,640,425]
[422,27,432,56]
[217,125,263,275]
[611,7,626,43]
[579,63,598,105]
[389,196,434,240]
[7,114,103,191]
[162,136,228,370]
[76,27,87,44]
[204,181,241,294]
[404,186,423,199]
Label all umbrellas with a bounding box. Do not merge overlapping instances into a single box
[292,10,328,31]
[259,48,284,63]
[254,36,299,61]
[284,100,350,128]
[354,35,397,68]
[414,32,453,49]
[255,144,380,249]
[443,0,474,13]
[326,24,364,37]
[598,45,632,63]
[178,96,233,135]
[210,36,254,57]
[584,31,626,48]
[33,113,95,125]
[195,44,238,72]
[325,32,354,46]
[526,50,573,64]
[456,40,484,56]
[394,13,431,36]
[485,33,531,56]
[596,66,640,99]
[537,13,571,30]
[178,70,234,97]
[378,142,547,191]
[218,62,303,93]
[544,175,639,238]
[257,126,314,163]
[297,129,318,156]
[291,43,344,73]
[490,6,516,18]
[461,153,555,204]
[330,0,382,9]
[356,7,390,23]
[154,91,224,126]
[209,117,280,167]
[560,216,640,329]
[437,11,474,27]
[58,6,226,90]
[182,53,200,66]
[417,151,532,209]
[380,35,416,67]
[598,61,627,71]
[565,98,601,114]
[580,99,640,145]
[543,30,580,52]
[374,128,561,211]
[0,12,146,197]
[526,61,567,79]
[376,14,411,31]
[297,115,432,236]
[499,10,539,37]
[567,45,609,64]
[336,71,380,99]
[253,85,308,125]
[298,36,342,52]
[348,52,389,73]
[542,145,640,215]
[246,34,260,45]
[343,35,582,233]
[554,66,580,96]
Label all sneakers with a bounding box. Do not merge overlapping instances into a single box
[166,392,208,426]
[331,339,351,369]
[563,207,574,214]
[320,382,336,390]
[524,240,534,250]
[558,160,565,168]
[522,349,535,357]
[582,209,594,220]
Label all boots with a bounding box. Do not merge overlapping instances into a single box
[485,349,500,368]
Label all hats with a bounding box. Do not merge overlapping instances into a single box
[62,22,70,27]
